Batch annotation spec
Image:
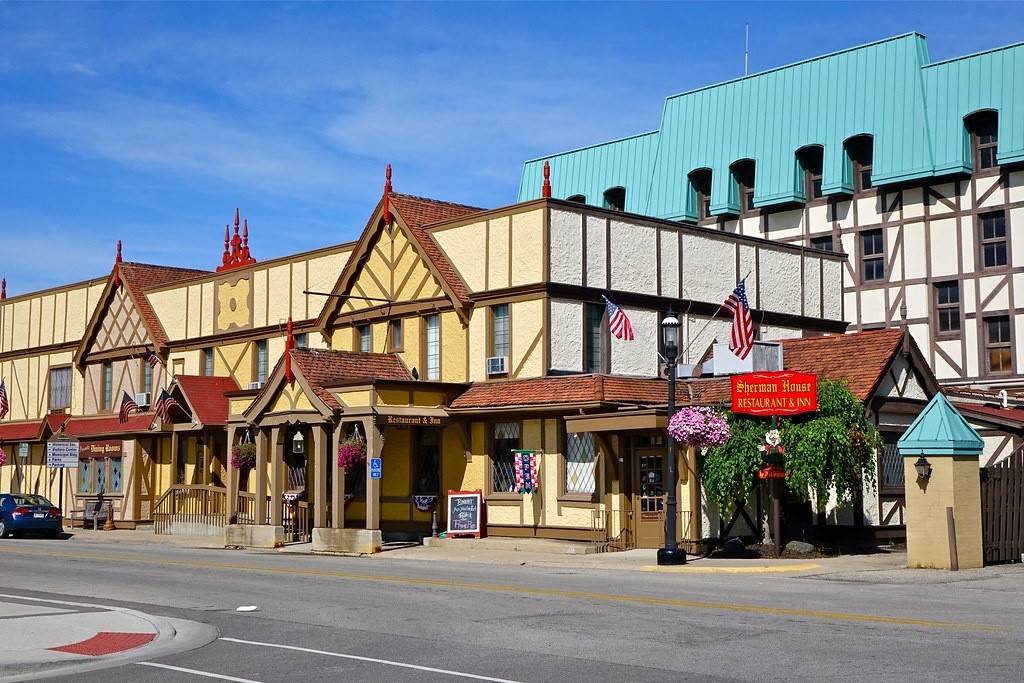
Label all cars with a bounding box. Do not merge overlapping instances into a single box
[0,492,64,540]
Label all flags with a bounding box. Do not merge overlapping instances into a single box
[605,298,634,341]
[119,393,138,424]
[156,390,178,424]
[720,280,754,359]
[146,347,159,369]
[0,382,9,420]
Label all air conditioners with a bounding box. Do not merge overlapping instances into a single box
[135,391,151,407]
[486,356,509,375]
[250,381,267,390]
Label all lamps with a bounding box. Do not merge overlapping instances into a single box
[292,430,308,454]
[913,450,933,483]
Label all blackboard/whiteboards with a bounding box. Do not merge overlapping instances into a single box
[447,493,481,534]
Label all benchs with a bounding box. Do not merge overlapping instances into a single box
[68,500,114,531]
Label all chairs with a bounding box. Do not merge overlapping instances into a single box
[2,498,17,507]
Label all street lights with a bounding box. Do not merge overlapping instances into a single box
[654,307,692,565]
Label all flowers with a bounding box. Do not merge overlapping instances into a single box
[229,442,256,472]
[336,439,367,468]
[665,407,730,447]
